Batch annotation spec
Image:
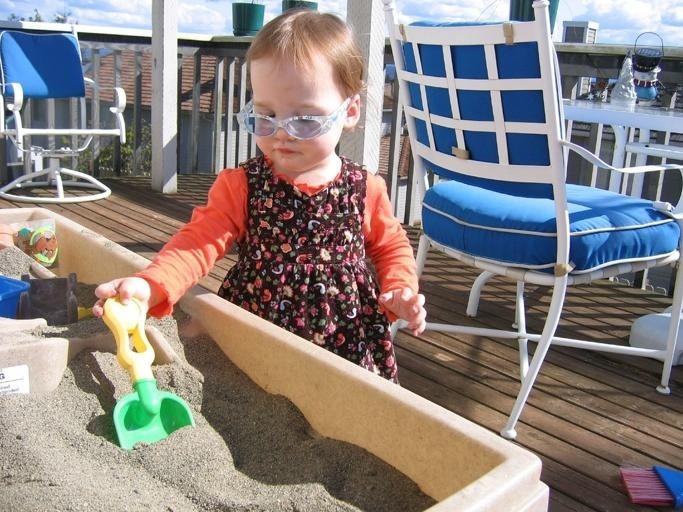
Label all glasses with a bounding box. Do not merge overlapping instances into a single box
[236,97,352,141]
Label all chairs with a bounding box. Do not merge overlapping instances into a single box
[0,23,127,203]
[380,0,683,439]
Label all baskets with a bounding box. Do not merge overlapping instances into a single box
[633,31,665,72]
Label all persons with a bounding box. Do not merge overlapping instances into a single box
[92,8,429,388]
